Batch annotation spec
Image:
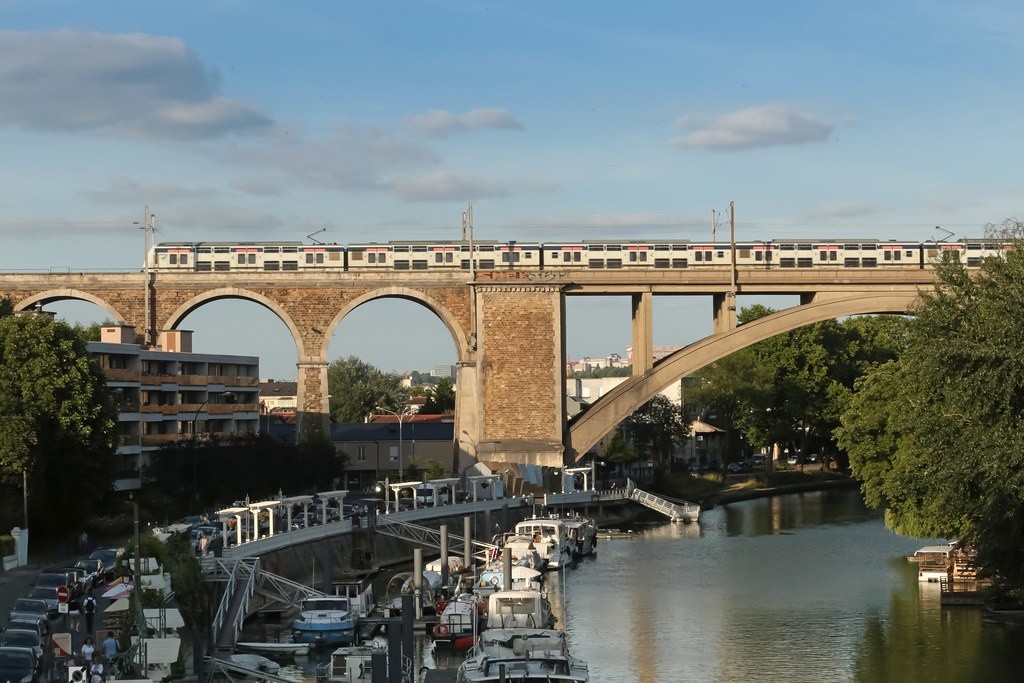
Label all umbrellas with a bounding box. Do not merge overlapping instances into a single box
[101,575,135,614]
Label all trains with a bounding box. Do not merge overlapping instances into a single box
[141,239,1021,271]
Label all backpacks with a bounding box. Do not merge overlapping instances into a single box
[85,596,95,613]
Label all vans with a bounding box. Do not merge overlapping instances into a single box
[417,483,449,506]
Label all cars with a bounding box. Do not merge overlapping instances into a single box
[8,597,48,629]
[0,615,47,641]
[788,456,799,465]
[806,454,820,463]
[0,647,40,683]
[731,463,746,474]
[0,628,43,658]
[754,454,765,464]
[28,548,119,619]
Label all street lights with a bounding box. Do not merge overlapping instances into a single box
[298,395,333,490]
[376,407,420,483]
[192,392,232,500]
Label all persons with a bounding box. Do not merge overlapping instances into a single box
[80,638,96,668]
[68,595,83,634]
[102,632,122,667]
[85,588,96,600]
[89,656,105,683]
[83,592,97,634]
[198,533,209,556]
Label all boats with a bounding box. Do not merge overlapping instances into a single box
[906,540,993,605]
[292,594,360,646]
[455,612,590,683]
[383,505,598,652]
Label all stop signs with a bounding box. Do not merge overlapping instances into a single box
[57,586,70,603]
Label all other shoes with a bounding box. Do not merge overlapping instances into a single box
[76,629,79,633]
[87,630,93,634]
